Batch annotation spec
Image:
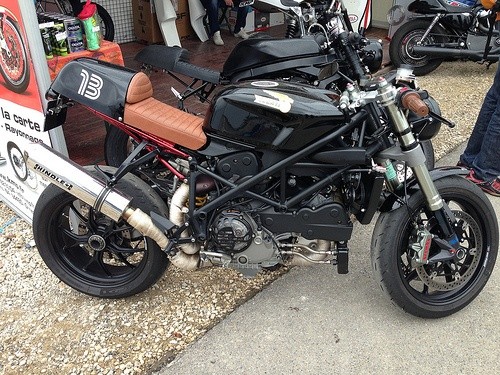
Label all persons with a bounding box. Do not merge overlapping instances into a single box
[456,61,500,198]
[200,0,250,45]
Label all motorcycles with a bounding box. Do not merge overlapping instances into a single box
[388,1,500,77]
[21,0,500,321]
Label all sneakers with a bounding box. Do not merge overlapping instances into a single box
[466,169,500,197]
[234,27,250,39]
[213,31,225,45]
